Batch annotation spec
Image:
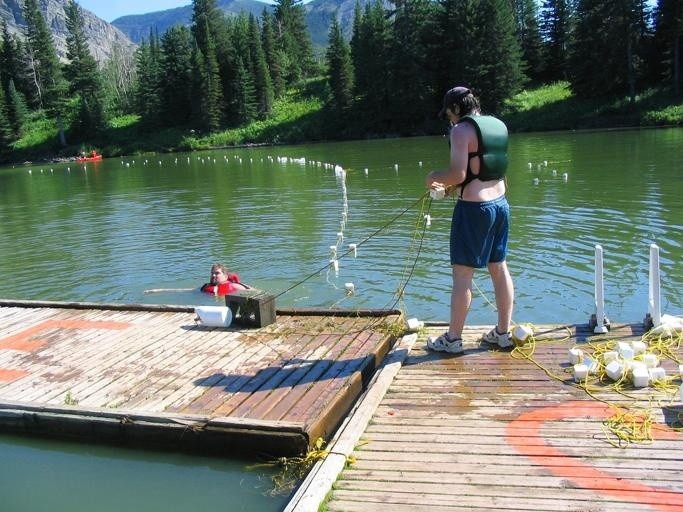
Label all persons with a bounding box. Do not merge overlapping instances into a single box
[90,150,96,158]
[426,86,516,355]
[142,262,261,297]
[81,151,87,158]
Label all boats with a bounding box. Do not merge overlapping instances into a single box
[79,154,102,161]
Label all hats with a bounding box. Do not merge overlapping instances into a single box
[438,86,470,116]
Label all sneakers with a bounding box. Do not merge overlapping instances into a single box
[482,325,514,349]
[426,332,464,354]
